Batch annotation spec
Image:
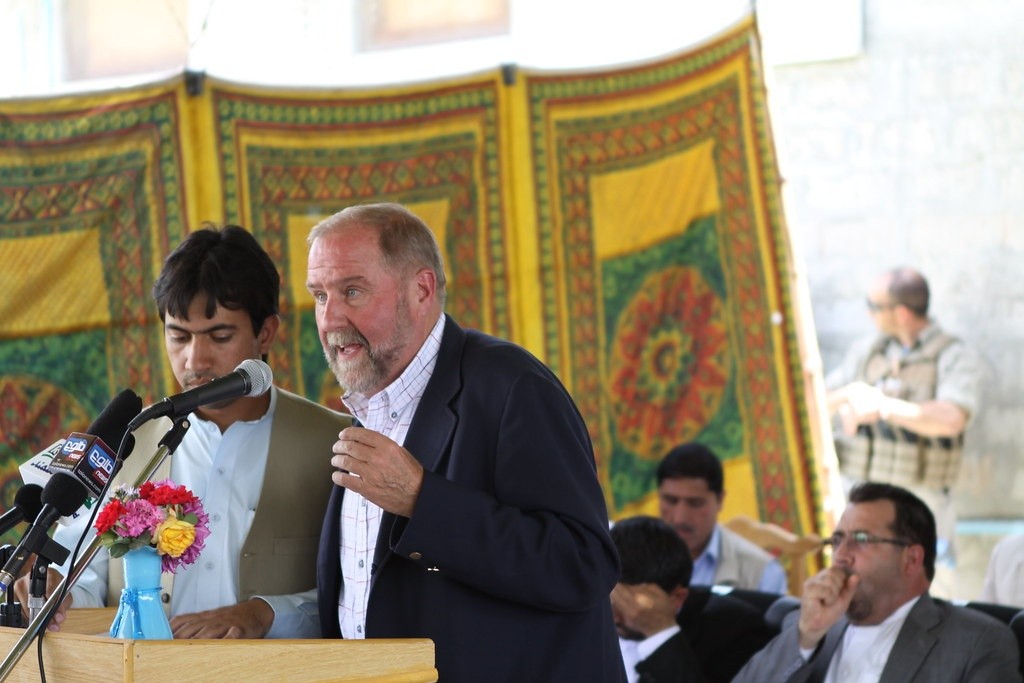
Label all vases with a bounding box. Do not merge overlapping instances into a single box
[109,546,174,640]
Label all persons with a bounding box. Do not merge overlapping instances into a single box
[11,220,355,642]
[306,203,630,682]
[610,268,1024,682]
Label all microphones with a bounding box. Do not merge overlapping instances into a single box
[129,359,274,430]
[0,390,142,597]
[0,433,135,537]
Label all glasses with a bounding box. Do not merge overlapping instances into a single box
[831,530,913,550]
[868,297,898,311]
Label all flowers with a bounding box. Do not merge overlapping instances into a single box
[93,478,211,574]
